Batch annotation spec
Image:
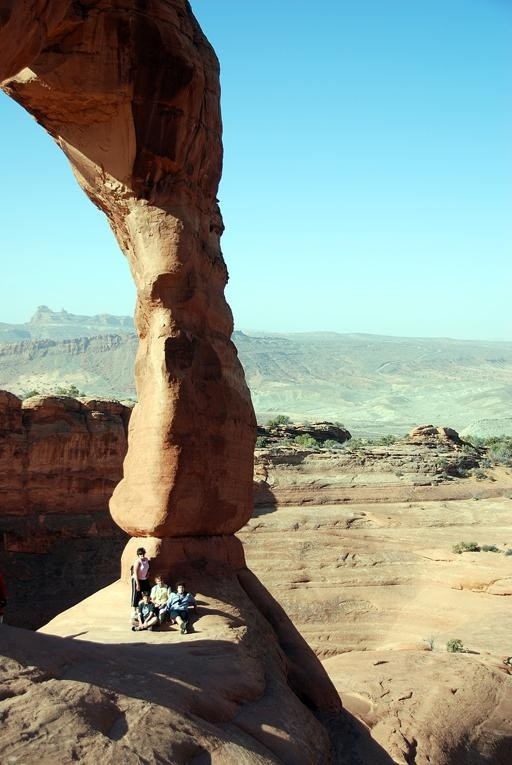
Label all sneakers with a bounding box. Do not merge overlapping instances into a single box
[132,626,152,632]
[180,622,189,634]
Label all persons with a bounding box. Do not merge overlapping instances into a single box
[169,580,202,634]
[131,546,151,610]
[150,574,171,626]
[132,589,159,631]
